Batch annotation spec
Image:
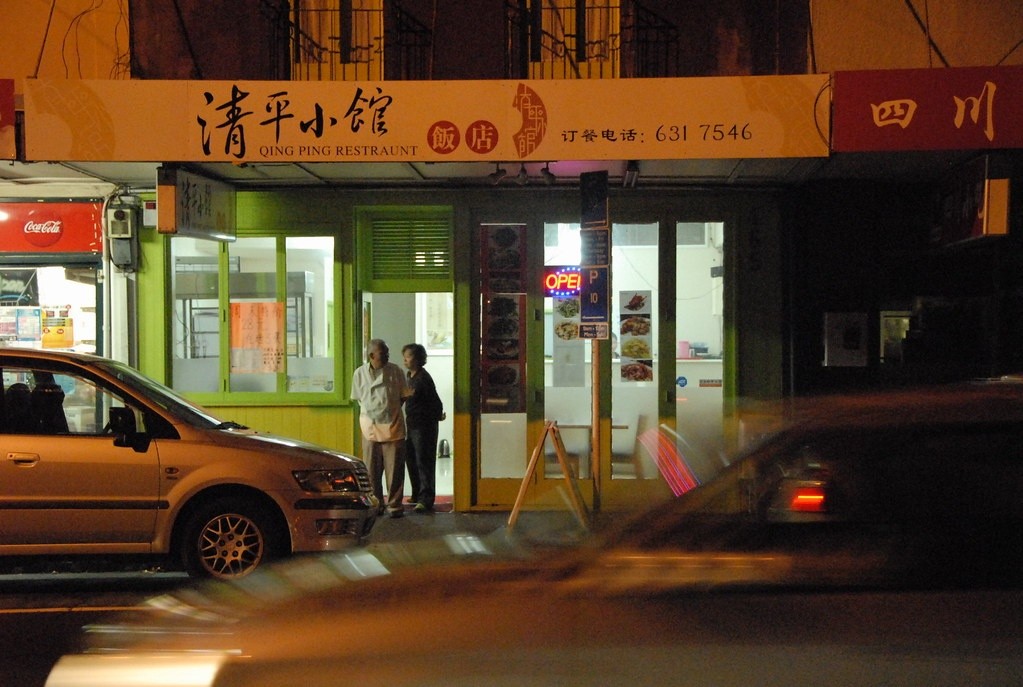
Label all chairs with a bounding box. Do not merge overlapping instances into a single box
[0,383,69,435]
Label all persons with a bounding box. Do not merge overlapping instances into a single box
[401,343,443,512]
[351,339,407,516]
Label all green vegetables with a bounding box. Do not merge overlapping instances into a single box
[487,295,519,336]
[557,299,579,318]
[489,227,519,269]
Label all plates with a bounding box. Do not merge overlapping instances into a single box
[620,317,651,337]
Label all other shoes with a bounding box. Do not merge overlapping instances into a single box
[407,498,416,503]
[414,503,426,511]
[379,506,384,515]
[389,508,403,517]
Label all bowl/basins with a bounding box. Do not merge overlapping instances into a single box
[553,318,579,341]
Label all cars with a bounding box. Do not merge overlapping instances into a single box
[28,370,1023,687]
[0,343,383,588]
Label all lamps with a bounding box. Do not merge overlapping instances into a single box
[513,161,529,185]
[540,161,557,185]
[487,163,506,185]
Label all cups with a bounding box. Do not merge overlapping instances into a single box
[677,339,689,358]
